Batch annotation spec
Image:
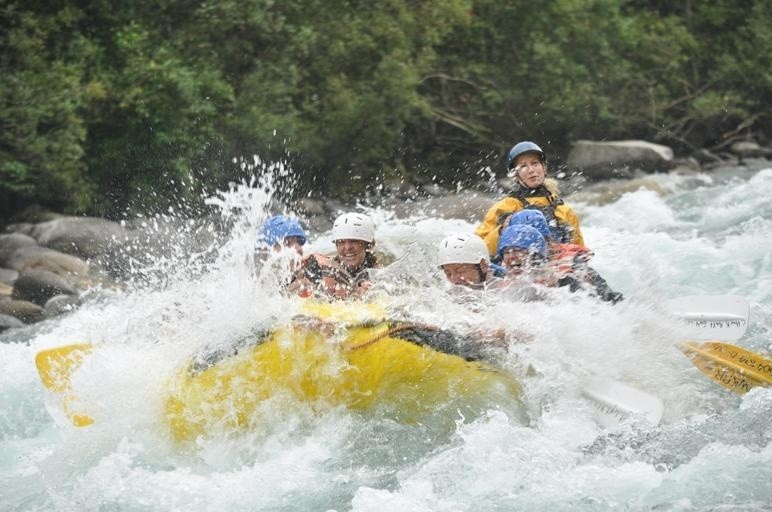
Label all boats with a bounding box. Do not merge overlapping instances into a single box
[157,315,528,450]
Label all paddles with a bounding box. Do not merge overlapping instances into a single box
[659,295,771,396]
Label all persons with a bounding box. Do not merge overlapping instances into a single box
[436,230,491,290]
[283,210,386,302]
[246,214,307,280]
[489,223,624,306]
[478,139,585,255]
[507,208,590,263]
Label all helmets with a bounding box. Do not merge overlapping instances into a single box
[511,208,552,237]
[327,210,376,245]
[432,233,494,269]
[257,214,306,247]
[495,224,552,260]
[506,141,550,168]
